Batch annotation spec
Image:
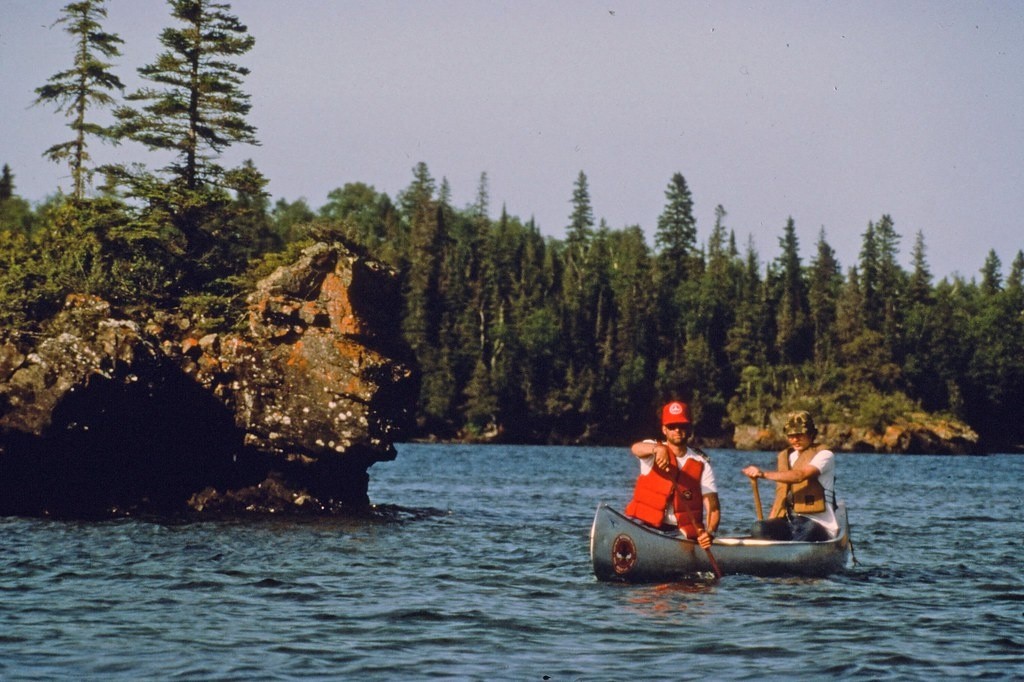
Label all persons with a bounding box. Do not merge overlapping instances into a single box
[626,401,721,549]
[742,410,839,541]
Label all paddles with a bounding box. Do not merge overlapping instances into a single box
[670,474,724,577]
[749,477,764,522]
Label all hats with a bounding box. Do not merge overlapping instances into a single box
[662,401,692,426]
[785,410,815,435]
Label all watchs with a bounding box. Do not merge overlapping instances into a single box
[710,532,716,538]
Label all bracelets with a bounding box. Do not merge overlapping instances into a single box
[761,471,764,478]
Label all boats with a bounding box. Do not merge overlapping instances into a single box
[589,499,850,586]
